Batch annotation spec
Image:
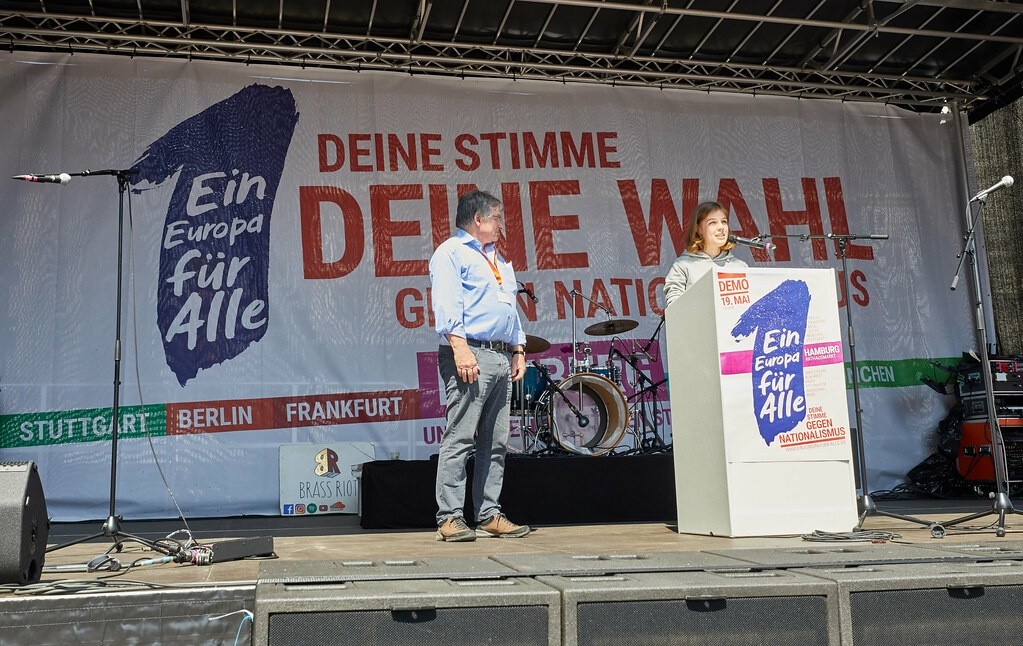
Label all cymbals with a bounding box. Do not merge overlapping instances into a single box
[508,335,551,353]
[584,320,639,336]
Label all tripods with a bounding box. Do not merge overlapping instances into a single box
[27,170,178,553]
[629,357,666,455]
[506,352,583,454]
[754,195,1023,536]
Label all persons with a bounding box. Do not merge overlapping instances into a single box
[428,189,530,542]
[662,202,748,307]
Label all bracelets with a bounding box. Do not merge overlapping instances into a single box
[512,351,525,357]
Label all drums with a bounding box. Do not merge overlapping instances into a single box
[510,365,551,417]
[536,373,629,456]
[571,366,621,387]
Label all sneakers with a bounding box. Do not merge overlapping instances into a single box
[475,512,530,538]
[436,516,476,542]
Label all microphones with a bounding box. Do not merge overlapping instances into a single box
[608,338,615,362]
[728,235,776,251]
[521,283,539,303]
[636,341,655,362]
[13,173,71,185]
[576,413,589,427]
[970,176,1014,202]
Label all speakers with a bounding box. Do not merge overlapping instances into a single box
[0,460,50,586]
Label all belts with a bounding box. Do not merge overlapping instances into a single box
[467,338,509,350]
